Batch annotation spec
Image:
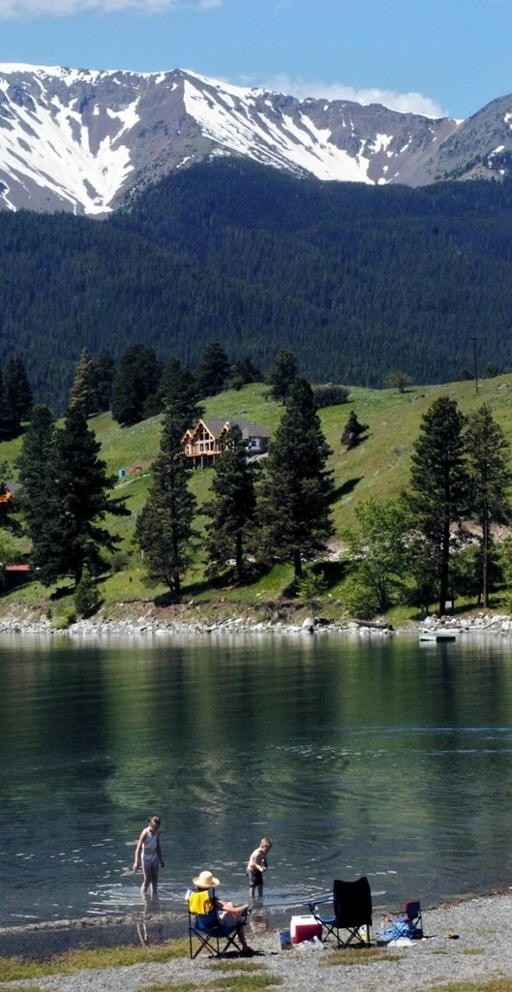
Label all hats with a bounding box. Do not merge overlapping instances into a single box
[192,871,220,889]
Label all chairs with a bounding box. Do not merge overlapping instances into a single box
[303,876,372,948]
[389,901,423,938]
[186,889,247,958]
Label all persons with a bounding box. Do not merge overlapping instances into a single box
[247,898,271,934]
[192,871,258,954]
[247,838,272,898]
[136,894,163,948]
[132,816,165,893]
